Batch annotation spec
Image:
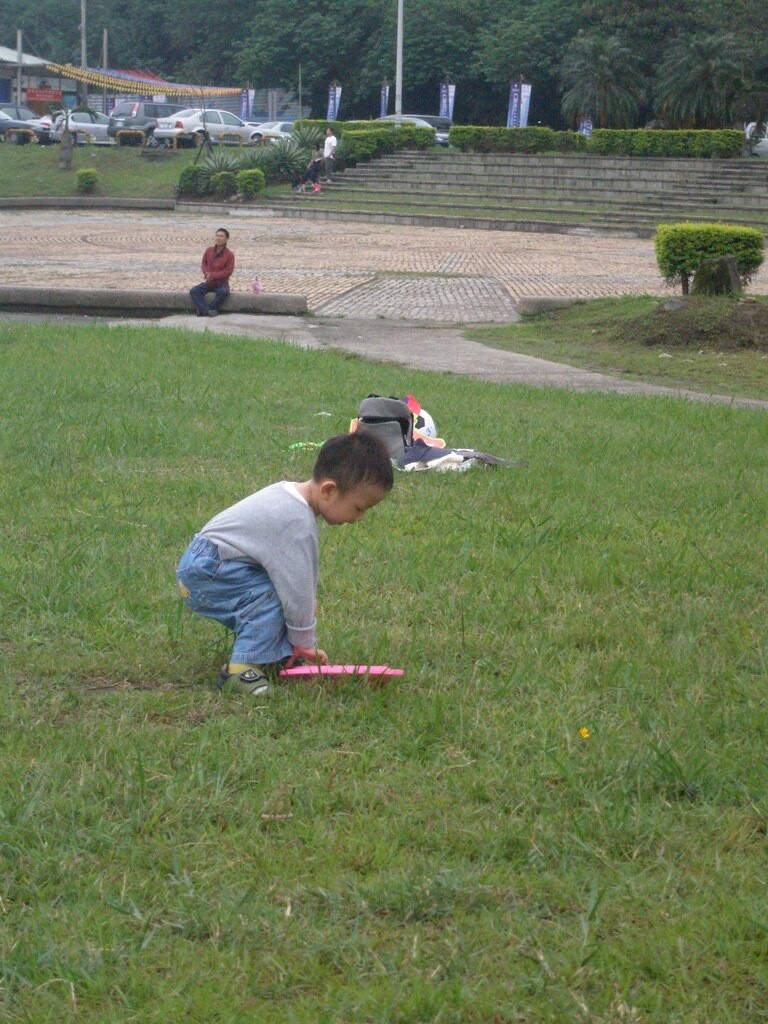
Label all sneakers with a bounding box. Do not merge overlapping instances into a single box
[262,657,303,669]
[314,183,320,192]
[216,664,271,696]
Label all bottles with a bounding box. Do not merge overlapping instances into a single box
[252,276,260,294]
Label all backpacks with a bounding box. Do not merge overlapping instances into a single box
[357,393,414,459]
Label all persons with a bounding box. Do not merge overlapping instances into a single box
[190,228,234,316]
[175,432,395,698]
[307,127,337,192]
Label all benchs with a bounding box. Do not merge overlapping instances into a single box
[0,284,309,314]
[0,197,176,210]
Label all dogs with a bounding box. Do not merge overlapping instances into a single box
[292,171,315,192]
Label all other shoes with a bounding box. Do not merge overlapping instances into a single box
[327,179,332,182]
[208,309,217,316]
[198,305,208,315]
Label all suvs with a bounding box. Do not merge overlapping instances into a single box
[106,100,194,149]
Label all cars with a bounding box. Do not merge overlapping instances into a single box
[0,102,50,145]
[26,108,116,145]
[377,113,454,147]
[744,120,768,157]
[248,121,296,143]
[153,109,264,148]
